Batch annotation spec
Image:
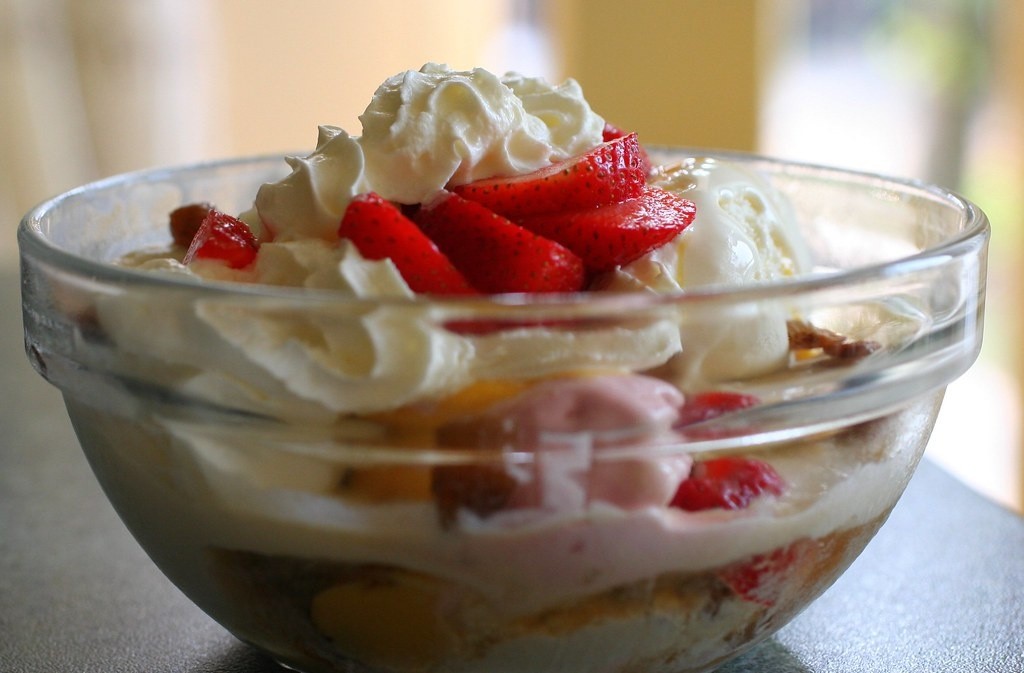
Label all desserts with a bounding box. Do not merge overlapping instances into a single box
[29,60,947,673]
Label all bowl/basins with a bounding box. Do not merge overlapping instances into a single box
[16,153,990,672]
